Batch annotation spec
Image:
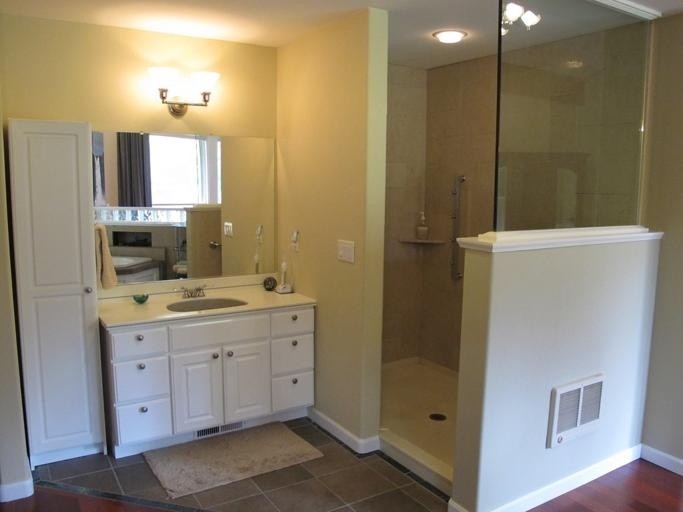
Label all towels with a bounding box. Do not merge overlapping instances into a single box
[94,221,118,291]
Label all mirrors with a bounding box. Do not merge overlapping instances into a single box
[89,133,276,292]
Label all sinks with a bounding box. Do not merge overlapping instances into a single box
[166,298,248,312]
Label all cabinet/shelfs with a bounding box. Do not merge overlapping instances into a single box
[5,115,110,473]
[97,305,318,458]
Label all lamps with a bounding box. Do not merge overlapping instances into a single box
[501,1,540,41]
[147,66,219,119]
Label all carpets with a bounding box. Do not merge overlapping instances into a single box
[136,424,325,500]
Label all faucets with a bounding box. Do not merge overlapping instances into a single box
[193,285,202,298]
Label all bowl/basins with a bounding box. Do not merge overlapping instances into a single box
[134,295,148,304]
